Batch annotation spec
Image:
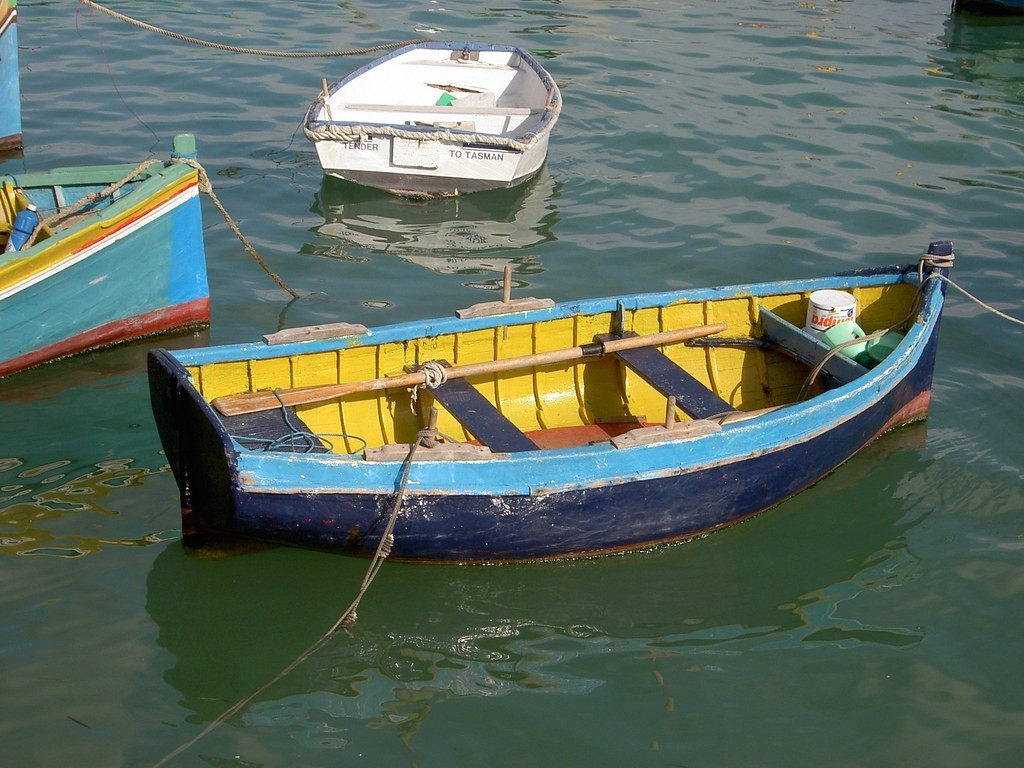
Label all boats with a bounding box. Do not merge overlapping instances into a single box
[147,240,955,566]
[0,0,26,150]
[0,129,211,376]
[303,39,562,202]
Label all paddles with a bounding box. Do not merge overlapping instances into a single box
[212,323,728,416]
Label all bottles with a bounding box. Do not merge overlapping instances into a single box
[5,204,38,252]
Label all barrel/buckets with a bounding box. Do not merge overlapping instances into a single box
[804,290,857,338]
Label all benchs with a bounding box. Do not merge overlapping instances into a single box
[346,101,541,121]
[403,59,524,72]
[208,386,333,455]
[404,359,541,453]
[593,330,738,419]
